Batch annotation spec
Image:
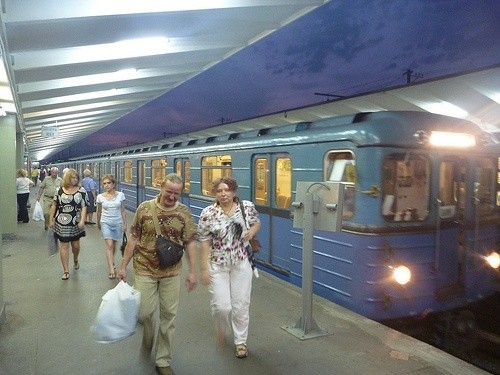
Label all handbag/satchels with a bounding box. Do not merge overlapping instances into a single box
[155,236,184,269]
[48,228,58,257]
[95,280,140,345]
[32,200,45,221]
[250,237,262,252]
[121,233,127,257]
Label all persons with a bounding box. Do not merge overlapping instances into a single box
[16,169,35,223]
[96,175,127,280]
[197,177,261,357]
[35,167,62,230]
[118,173,199,375]
[31,166,51,184]
[81,169,97,225]
[49,168,89,279]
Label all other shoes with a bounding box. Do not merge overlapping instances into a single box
[61,271,69,280]
[109,272,116,279]
[235,344,248,358]
[156,365,174,375]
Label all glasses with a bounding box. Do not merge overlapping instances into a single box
[102,182,110,185]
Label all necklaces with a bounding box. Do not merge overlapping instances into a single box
[227,203,233,216]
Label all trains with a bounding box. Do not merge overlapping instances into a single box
[40,109,500,323]
